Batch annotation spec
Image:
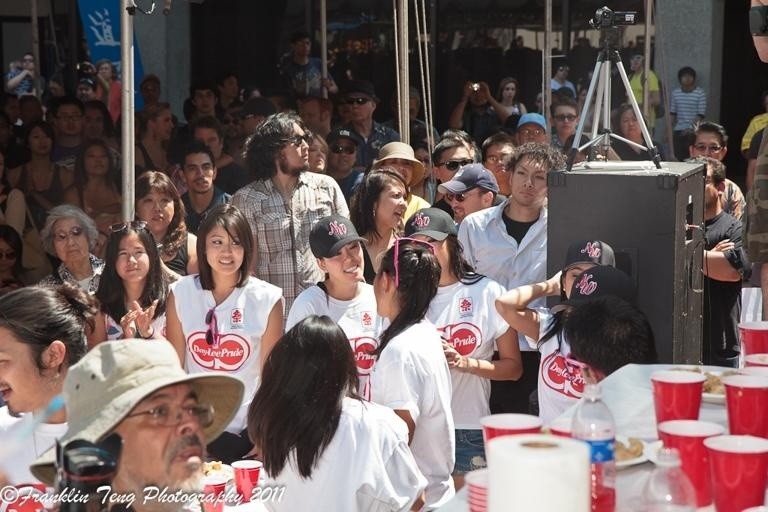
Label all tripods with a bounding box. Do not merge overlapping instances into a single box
[566,27,662,172]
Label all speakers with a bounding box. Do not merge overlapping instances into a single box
[546,160,707,366]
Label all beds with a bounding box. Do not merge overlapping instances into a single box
[433,362,768,512]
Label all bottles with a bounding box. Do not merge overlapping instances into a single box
[642,449,698,510]
[574,385,616,511]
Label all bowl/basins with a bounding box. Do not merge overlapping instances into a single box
[649,321,767,512]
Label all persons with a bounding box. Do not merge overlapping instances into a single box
[0,0,767,512]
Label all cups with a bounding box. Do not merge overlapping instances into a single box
[464,471,490,510]
[190,470,229,511]
[478,415,543,437]
[551,417,607,486]
[230,460,261,500]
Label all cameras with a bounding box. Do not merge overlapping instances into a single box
[54,430,124,512]
[588,6,637,30]
[76,63,88,70]
[469,83,481,92]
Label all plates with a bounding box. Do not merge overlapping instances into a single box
[617,434,654,470]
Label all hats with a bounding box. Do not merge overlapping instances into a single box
[29,337,244,488]
